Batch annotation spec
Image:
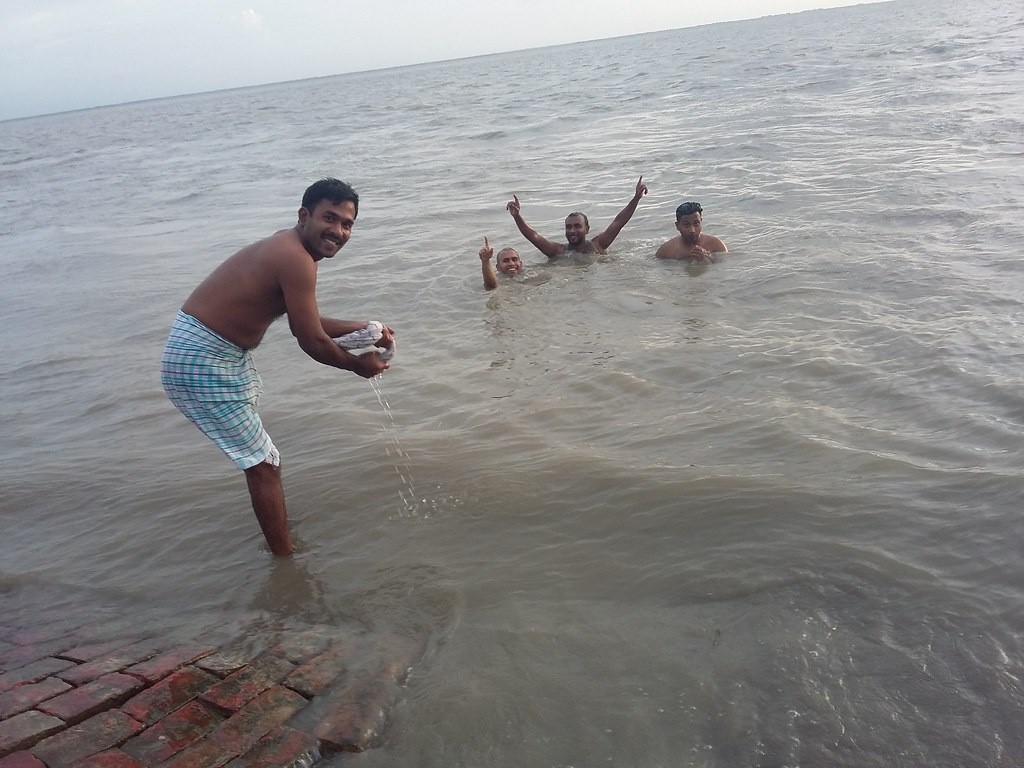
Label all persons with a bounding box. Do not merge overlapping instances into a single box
[654,202,728,264]
[479,236,523,291]
[506,175,648,260]
[159,176,394,559]
